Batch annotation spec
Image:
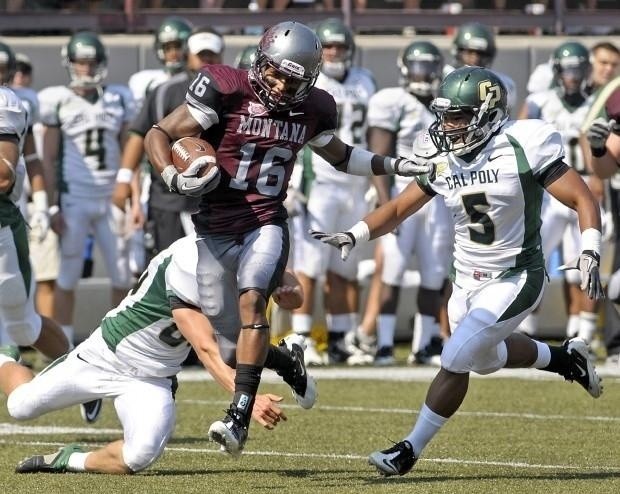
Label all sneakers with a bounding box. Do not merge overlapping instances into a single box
[80,399,102,423]
[278,333,318,410]
[14,445,81,473]
[370,441,418,476]
[564,339,603,398]
[407,353,442,367]
[210,409,248,452]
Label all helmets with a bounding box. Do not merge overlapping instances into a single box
[553,42,591,88]
[398,23,509,157]
[239,18,354,114]
[155,18,192,72]
[59,31,107,88]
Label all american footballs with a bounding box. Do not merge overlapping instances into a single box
[171,137,220,178]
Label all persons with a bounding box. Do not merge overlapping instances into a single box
[0,0,620,477]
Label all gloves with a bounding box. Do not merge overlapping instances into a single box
[29,191,50,241]
[585,118,617,157]
[558,228,606,302]
[161,161,220,197]
[308,221,370,260]
[383,154,432,176]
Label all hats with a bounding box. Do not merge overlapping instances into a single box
[185,26,223,58]
